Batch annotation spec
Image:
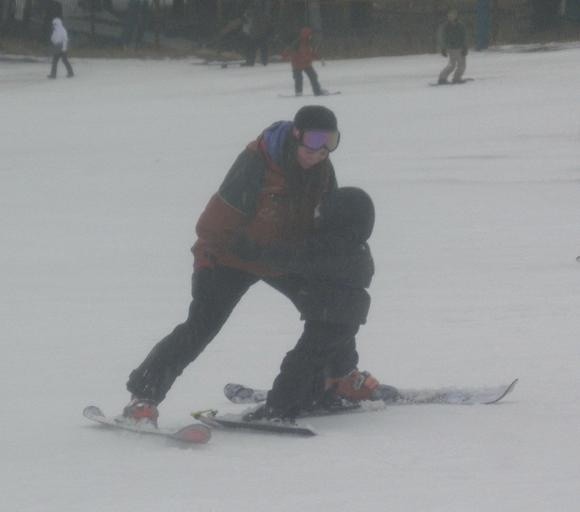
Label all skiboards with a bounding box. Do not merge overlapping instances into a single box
[189,399,385,434]
[81,377,517,440]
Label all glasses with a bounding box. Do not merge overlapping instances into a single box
[294,124,340,155]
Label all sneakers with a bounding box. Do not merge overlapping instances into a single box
[322,367,399,404]
[122,391,162,424]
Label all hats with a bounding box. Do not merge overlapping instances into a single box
[315,184,376,248]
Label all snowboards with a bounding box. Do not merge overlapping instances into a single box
[429,79,474,86]
[277,92,344,97]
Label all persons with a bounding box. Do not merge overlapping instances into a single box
[276,25,328,97]
[46,17,73,81]
[437,8,468,85]
[239,0,271,67]
